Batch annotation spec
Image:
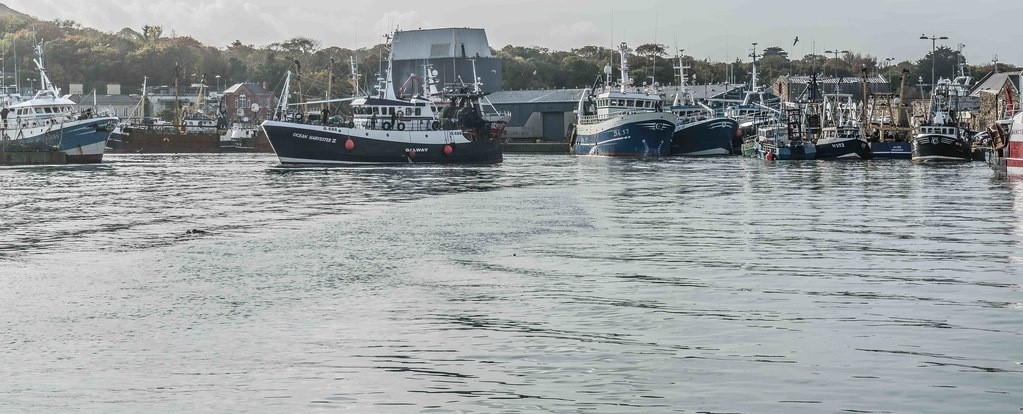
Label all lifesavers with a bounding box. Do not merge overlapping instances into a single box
[295,112,303,120]
[348,121,355,129]
[397,123,405,131]
[432,121,442,130]
[382,121,391,130]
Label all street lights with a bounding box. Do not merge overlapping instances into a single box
[824,50,849,103]
[920,35,948,98]
[885,57,895,95]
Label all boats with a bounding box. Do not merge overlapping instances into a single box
[1,37,120,166]
[565,39,1023,183]
[251,60,512,169]
